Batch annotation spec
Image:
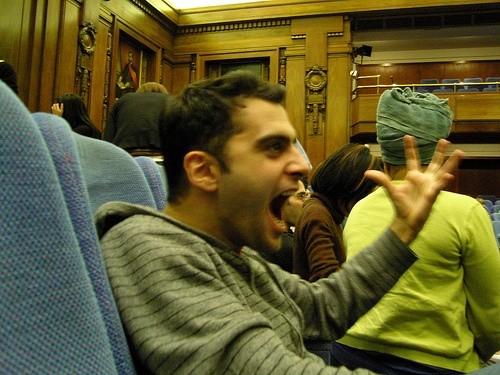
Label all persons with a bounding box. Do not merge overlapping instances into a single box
[331,85,500,375]
[291,141,383,283]
[93,71,463,375]
[52,93,101,139]
[102,82,171,158]
[260,180,306,274]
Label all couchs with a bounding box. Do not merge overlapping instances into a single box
[0,80,167,375]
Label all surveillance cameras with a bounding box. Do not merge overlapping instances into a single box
[352,44,372,57]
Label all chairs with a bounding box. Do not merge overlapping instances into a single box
[416,77,500,93]
